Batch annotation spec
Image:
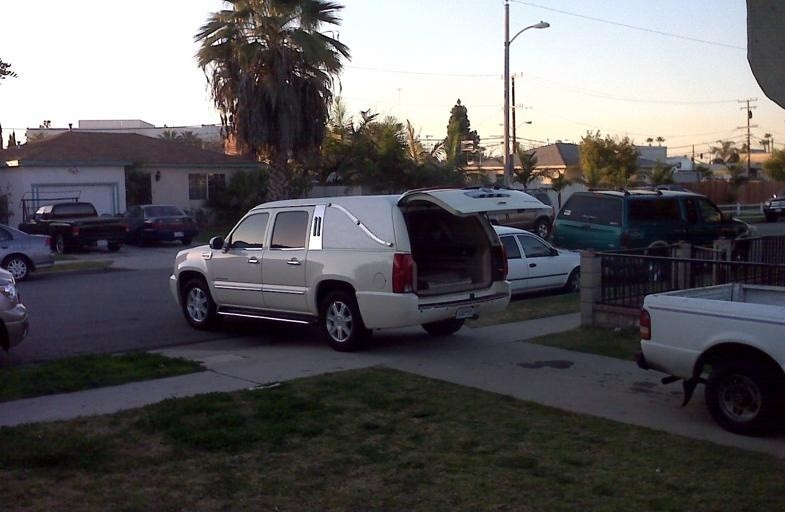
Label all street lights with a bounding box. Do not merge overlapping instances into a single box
[504,0,550,190]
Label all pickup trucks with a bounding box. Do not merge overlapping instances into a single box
[635,282,785,437]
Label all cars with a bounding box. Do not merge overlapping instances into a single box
[0,201,199,353]
